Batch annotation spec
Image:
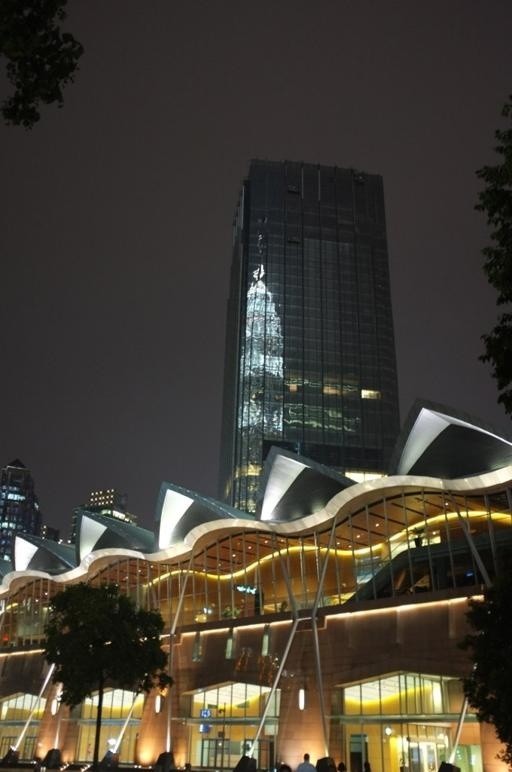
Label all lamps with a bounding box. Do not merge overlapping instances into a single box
[298,681,306,710]
[155,694,161,714]
[192,622,270,663]
[51,699,58,716]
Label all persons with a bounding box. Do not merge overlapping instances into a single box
[296,753,317,772]
[338,762,345,772]
[364,762,371,772]
[279,600,288,613]
